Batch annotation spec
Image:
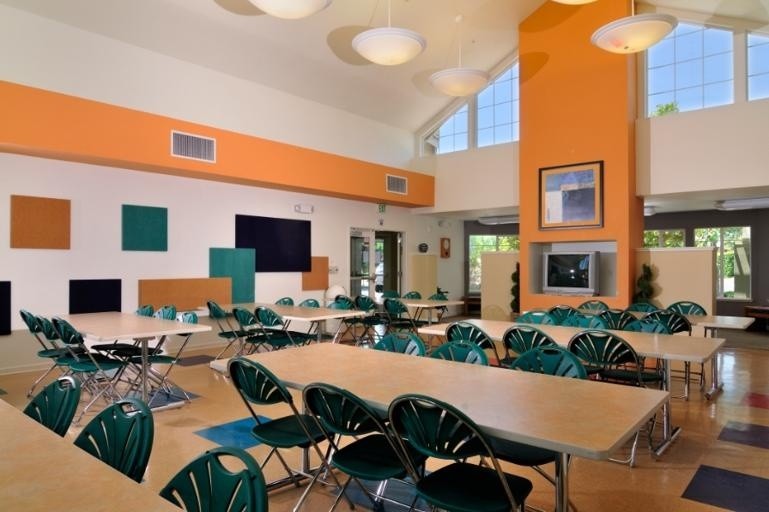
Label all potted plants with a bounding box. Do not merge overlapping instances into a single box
[509,262,519,322]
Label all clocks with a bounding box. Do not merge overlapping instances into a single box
[440,238,450,258]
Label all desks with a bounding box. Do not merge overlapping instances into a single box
[744,305,769,332]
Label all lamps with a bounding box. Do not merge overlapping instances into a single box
[351,0,427,67]
[248,0,335,21]
[588,0,679,55]
[429,12,491,99]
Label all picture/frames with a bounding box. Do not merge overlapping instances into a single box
[538,159,604,231]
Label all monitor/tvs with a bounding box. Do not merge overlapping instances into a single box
[541,252,602,296]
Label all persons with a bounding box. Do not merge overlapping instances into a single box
[374,253,384,292]
